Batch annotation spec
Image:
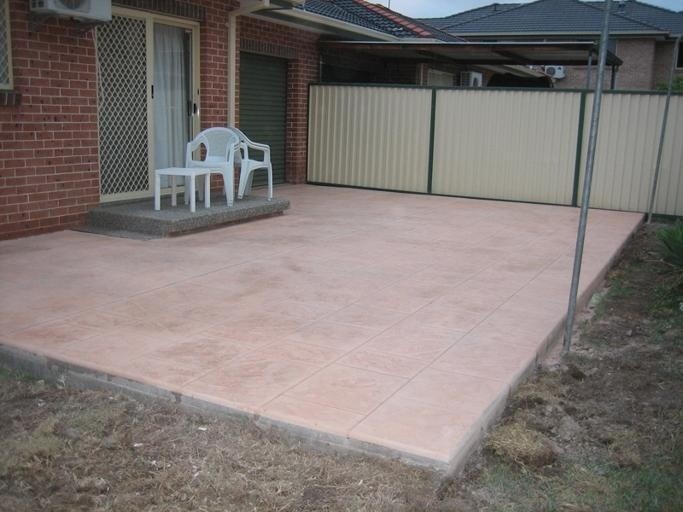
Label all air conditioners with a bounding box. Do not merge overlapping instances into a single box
[29,1,113,39]
[543,65,564,79]
[460,71,482,87]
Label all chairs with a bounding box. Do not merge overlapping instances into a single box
[151,126,273,213]
